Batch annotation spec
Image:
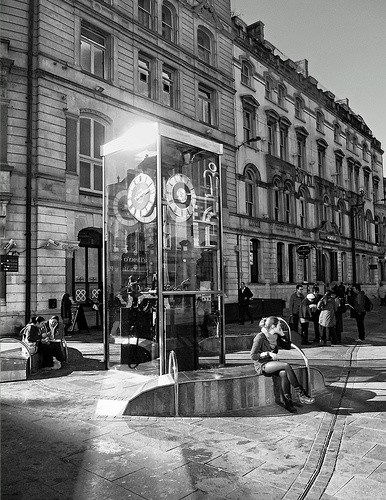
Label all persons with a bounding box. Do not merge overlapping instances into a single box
[238,282,253,325]
[19,316,65,371]
[289,282,366,347]
[250,316,315,413]
[377,282,386,306]
[61,293,72,338]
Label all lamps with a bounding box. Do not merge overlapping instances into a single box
[238,136,261,151]
[95,86,104,93]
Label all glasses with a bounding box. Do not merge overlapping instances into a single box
[298,289,303,291]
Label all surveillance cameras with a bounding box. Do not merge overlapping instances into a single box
[4,239,17,250]
[45,238,60,247]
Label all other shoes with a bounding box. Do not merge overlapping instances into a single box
[354,338,366,343]
[251,319,254,324]
[238,323,244,325]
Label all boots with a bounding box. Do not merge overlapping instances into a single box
[294,385,316,405]
[282,393,297,413]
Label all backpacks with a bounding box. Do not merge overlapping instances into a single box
[362,294,373,312]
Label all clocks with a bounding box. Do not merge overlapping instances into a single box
[166,173,197,217]
[128,174,156,217]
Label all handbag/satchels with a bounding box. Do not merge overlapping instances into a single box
[21,338,38,355]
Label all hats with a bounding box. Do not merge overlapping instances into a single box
[306,294,315,299]
